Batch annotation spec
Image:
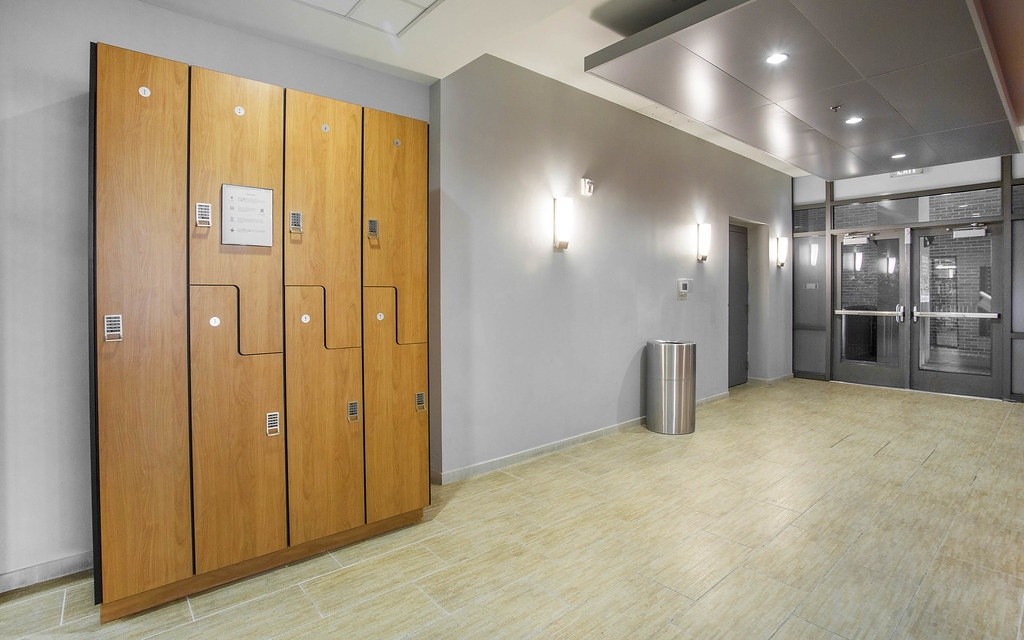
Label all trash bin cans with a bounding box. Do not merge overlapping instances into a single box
[646,339,697,435]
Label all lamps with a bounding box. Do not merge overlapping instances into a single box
[809,245,818,267]
[854,252,862,271]
[697,223,711,262]
[553,196,572,253]
[777,237,788,268]
[886,257,896,275]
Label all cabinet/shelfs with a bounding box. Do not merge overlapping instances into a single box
[88,41,431,623]
